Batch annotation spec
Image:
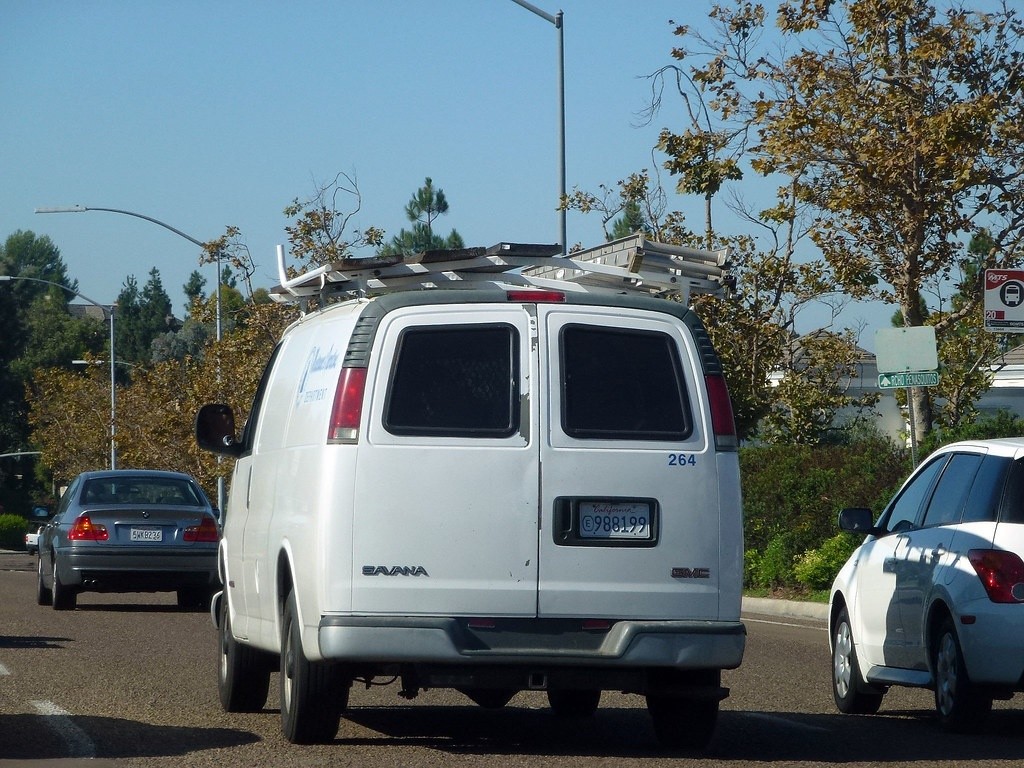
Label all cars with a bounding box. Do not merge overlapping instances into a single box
[36,469,222,613]
[826,439,1024,732]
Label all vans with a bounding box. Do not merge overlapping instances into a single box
[196,289,747,751]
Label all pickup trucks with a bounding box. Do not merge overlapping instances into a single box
[32,207,226,531]
[26,522,48,557]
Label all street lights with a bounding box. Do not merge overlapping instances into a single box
[1,275,119,496]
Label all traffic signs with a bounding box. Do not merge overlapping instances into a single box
[877,371,939,389]
